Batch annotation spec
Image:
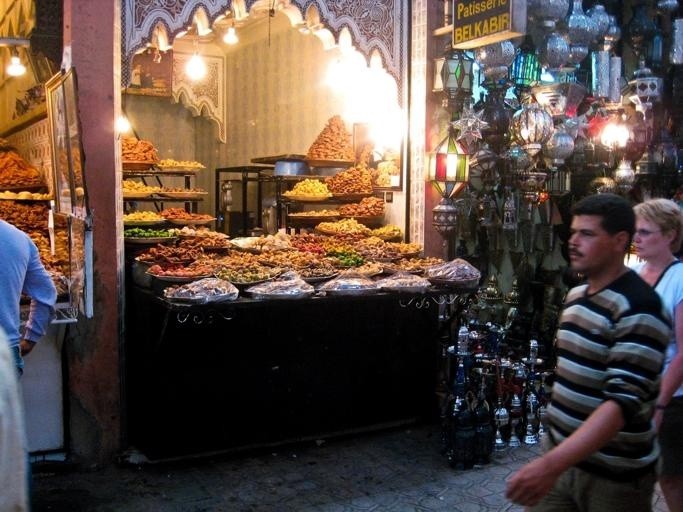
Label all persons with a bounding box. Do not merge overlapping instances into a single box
[631,198,682,512]
[503,193,675,511]
[0,215,58,511]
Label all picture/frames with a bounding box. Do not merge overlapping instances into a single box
[43,68,74,215]
[59,66,88,221]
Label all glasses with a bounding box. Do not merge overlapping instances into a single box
[634,228,665,236]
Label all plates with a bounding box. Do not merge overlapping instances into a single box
[304,157,354,168]
[134,236,481,310]
[283,194,386,228]
[120,161,219,243]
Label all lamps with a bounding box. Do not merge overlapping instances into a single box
[223,19,238,45]
[6,45,26,75]
[433,43,474,112]
[184,48,208,80]
[509,101,554,155]
[426,127,469,199]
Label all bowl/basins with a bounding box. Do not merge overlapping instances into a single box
[274,160,309,176]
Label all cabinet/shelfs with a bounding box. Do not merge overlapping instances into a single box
[122,154,479,473]
[0,146,80,471]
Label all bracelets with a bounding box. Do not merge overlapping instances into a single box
[656,402,670,412]
